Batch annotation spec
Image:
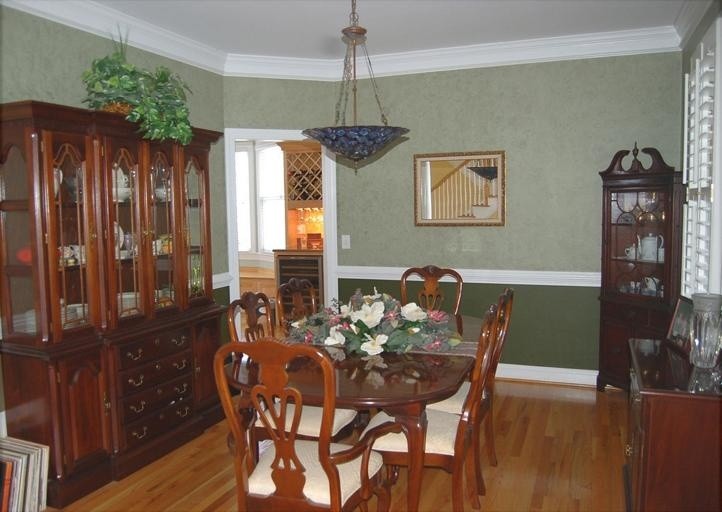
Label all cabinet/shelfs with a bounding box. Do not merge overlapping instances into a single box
[594,141,686,393]
[272,139,326,318]
[1,97,234,509]
[622,336,722,512]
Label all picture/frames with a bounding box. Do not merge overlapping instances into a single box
[664,293,695,352]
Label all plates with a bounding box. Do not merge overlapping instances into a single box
[114,219,125,251]
[13,291,146,333]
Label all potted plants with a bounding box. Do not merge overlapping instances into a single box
[80,19,196,147]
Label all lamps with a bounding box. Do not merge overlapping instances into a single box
[304,1,411,175]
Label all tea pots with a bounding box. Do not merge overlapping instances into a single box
[623,233,665,264]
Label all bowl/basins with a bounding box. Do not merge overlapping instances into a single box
[112,187,133,202]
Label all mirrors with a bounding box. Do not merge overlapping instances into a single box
[411,150,507,226]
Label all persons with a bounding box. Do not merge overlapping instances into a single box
[676,319,688,346]
[669,312,684,343]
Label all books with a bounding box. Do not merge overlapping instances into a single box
[0,435,53,512]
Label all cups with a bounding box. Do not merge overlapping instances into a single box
[689,292,721,368]
